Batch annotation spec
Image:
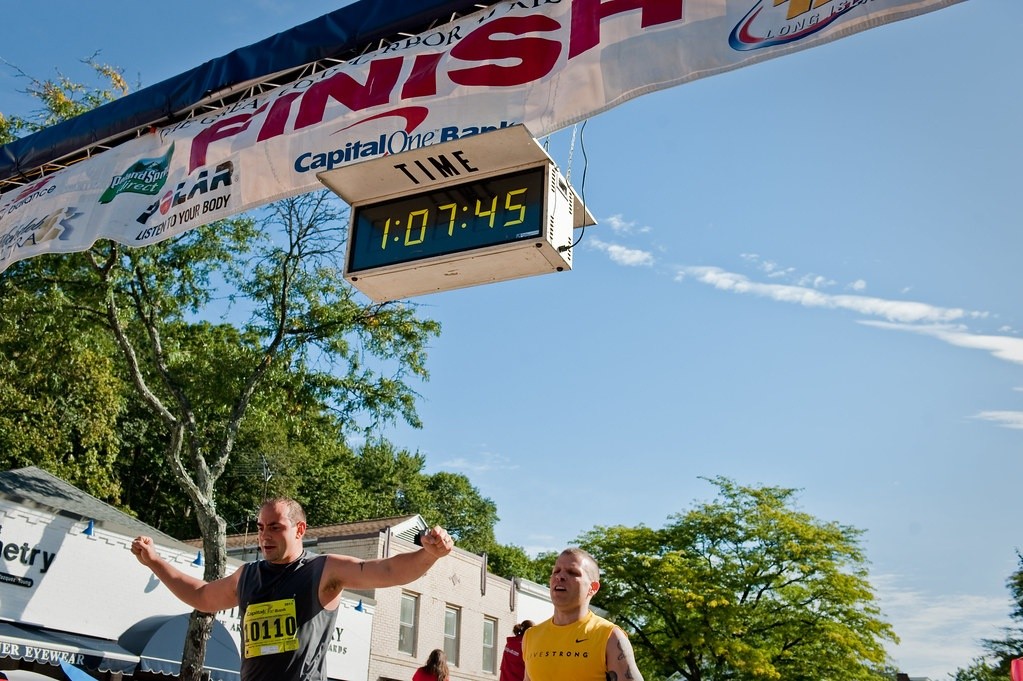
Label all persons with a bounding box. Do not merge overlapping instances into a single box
[130,498,455,681]
[499,620,533,681]
[522,548,644,681]
[412,649,452,681]
